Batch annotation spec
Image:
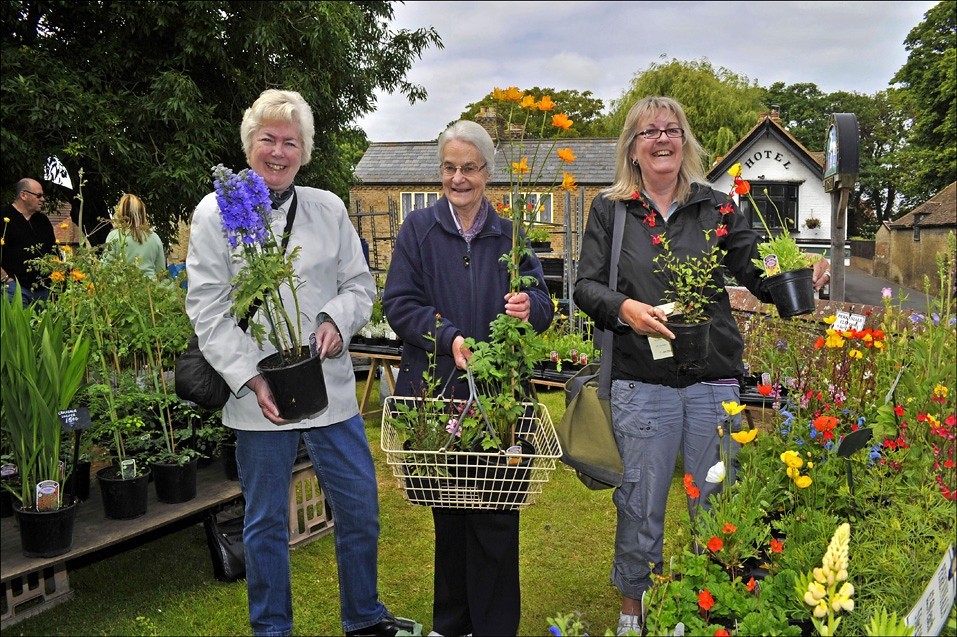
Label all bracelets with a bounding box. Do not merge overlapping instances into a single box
[1,274,9,283]
[321,319,344,344]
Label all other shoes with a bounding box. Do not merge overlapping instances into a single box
[346,611,419,637]
[427,631,472,637]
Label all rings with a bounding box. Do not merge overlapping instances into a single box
[825,271,830,277]
[653,306,657,316]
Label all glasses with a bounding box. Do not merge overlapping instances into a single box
[440,162,487,176]
[635,128,685,140]
[24,190,47,199]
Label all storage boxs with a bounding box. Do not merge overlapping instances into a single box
[1,562,74,631]
[382,395,562,509]
[288,465,336,551]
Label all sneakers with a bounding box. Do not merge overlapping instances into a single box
[617,612,641,637]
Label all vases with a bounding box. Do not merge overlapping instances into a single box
[530,242,554,253]
[762,268,816,321]
[403,436,464,487]
[148,451,197,503]
[0,483,24,519]
[661,315,712,362]
[97,464,151,520]
[64,460,89,502]
[401,460,440,504]
[256,345,330,420]
[472,435,538,508]
[220,435,241,481]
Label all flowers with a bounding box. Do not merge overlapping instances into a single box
[1,163,312,474]
[358,86,957,637]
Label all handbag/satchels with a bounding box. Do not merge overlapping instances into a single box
[558,358,624,490]
[173,335,230,409]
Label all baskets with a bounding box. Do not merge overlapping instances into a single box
[381,362,563,511]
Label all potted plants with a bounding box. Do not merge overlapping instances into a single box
[1,276,92,558]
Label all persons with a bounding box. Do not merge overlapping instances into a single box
[184,89,420,636]
[0,179,58,316]
[573,98,831,637]
[102,193,166,282]
[382,120,554,636]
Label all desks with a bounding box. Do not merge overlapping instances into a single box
[348,335,793,448]
